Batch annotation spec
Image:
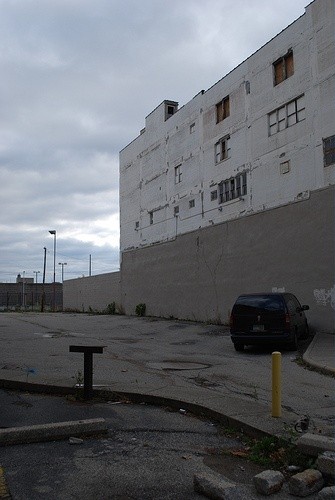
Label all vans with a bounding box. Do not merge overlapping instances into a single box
[229,293,310,351]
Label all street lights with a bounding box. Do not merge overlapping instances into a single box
[33,271,40,306]
[50,230,57,311]
[59,262,67,281]
